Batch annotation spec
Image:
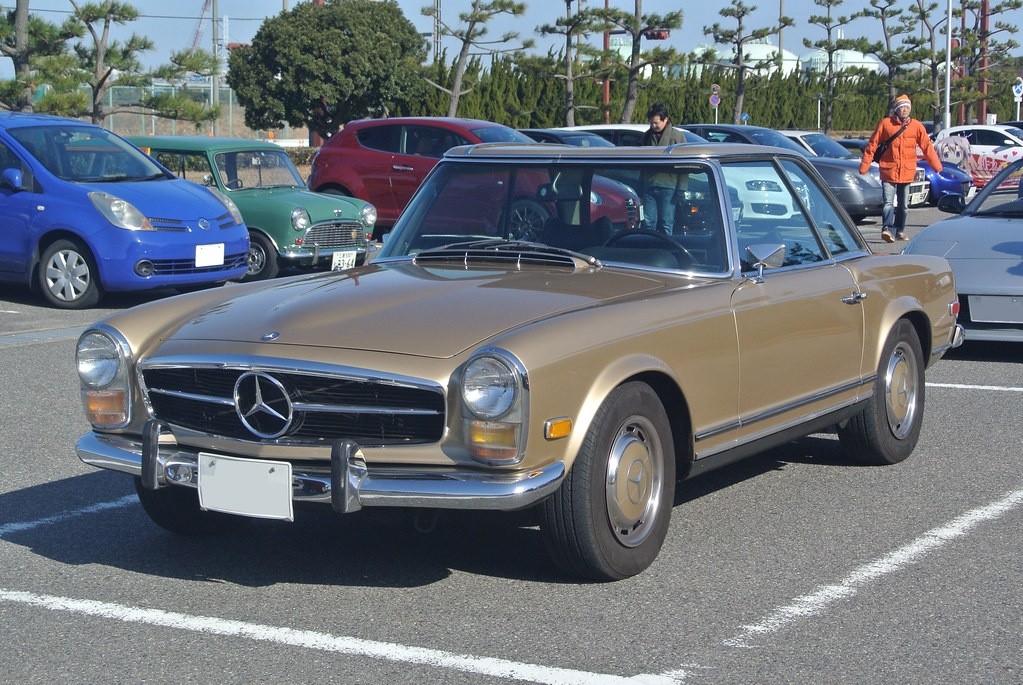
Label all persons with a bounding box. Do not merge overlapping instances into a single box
[859,92,942,243]
[637,102,688,237]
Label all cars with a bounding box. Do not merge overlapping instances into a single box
[998,121,1023,132]
[517,125,745,239]
[74,143,968,584]
[685,121,888,228]
[306,115,643,246]
[899,157,1023,344]
[65,131,377,283]
[920,120,947,142]
[0,110,250,311]
[931,124,1023,190]
[837,139,975,211]
[547,123,813,236]
[709,128,929,218]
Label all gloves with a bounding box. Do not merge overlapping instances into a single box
[671,190,686,209]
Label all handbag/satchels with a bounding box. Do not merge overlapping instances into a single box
[872,142,887,164]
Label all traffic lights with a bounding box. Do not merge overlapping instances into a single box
[645,31,670,41]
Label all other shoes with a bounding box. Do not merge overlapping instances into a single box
[881,231,895,242]
[896,229,909,241]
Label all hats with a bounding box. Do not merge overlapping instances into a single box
[894,94,912,112]
